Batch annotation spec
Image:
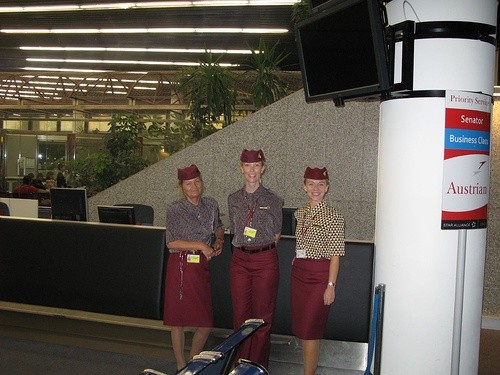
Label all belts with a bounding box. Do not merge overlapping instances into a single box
[237,241,276,253]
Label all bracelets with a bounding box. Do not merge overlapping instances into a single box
[328,282,336,288]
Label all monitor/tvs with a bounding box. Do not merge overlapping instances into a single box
[97,205,136,225]
[294,0,390,103]
[50,187,88,221]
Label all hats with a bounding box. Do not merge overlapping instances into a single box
[304,167,329,179]
[240,147,266,162]
[177,163,200,180]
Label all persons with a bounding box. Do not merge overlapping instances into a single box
[291,167,346,375]
[227,149,284,375]
[162,164,225,372]
[13,164,66,193]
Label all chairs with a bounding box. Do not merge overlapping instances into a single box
[115,203,154,226]
